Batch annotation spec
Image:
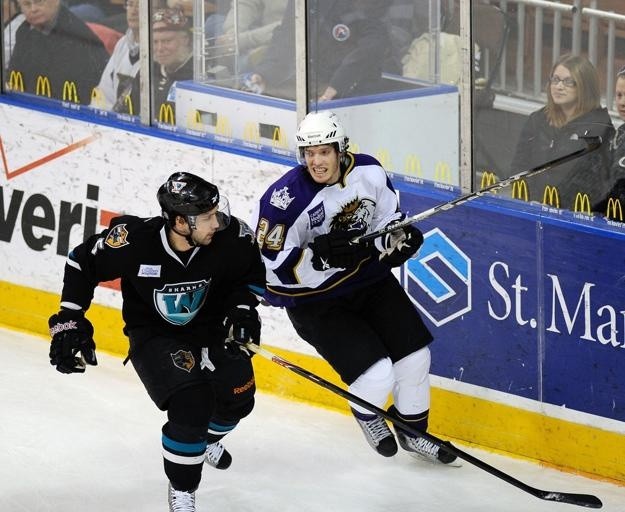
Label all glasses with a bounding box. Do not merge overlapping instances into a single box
[551,77,575,87]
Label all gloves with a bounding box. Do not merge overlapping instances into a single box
[224,292,261,359]
[375,220,422,268]
[48,308,97,373]
[307,226,371,271]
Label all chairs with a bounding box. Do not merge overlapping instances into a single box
[441,3,508,192]
[354,20,387,97]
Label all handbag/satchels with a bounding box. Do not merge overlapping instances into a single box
[401,32,481,87]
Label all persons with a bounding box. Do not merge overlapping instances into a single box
[253,108,459,464]
[552,68,625,222]
[508,54,615,224]
[47,170,267,512]
[0,0,416,125]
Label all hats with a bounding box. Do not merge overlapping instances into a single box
[151,7,190,31]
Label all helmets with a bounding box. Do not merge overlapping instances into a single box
[157,171,230,233]
[295,110,349,165]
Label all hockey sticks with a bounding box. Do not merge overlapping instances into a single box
[231,336,603,509]
[349,136,603,245]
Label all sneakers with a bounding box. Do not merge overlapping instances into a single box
[387,404,458,464]
[204,441,231,469]
[168,480,195,512]
[350,407,398,457]
